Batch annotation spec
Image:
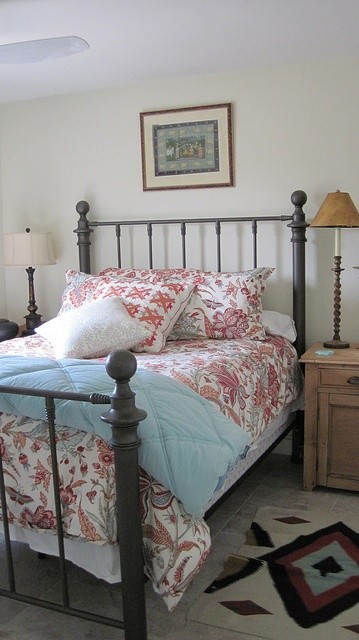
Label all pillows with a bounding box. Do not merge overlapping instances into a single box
[57,268,198,355]
[33,295,154,360]
[99,266,275,342]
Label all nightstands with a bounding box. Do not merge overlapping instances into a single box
[298,342,359,493]
[13,320,50,339]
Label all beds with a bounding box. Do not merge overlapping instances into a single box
[1,190,311,640]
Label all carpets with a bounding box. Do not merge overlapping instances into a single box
[182,505,358,640]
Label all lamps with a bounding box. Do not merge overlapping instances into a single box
[0,227,57,339]
[308,189,358,350]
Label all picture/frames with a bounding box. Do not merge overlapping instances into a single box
[138,101,235,193]
